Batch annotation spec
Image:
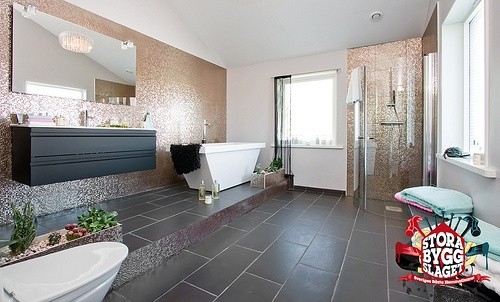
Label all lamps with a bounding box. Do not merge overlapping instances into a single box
[58,30,94,54]
[22,4,35,18]
[120,40,134,50]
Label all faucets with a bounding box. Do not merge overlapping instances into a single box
[201,120,210,141]
[84,110,93,127]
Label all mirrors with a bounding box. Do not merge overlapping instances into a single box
[11,3,137,108]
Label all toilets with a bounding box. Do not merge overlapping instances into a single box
[0,242,130,302]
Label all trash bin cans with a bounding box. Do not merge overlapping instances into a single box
[285,174,294,191]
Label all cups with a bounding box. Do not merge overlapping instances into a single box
[474,153,485,165]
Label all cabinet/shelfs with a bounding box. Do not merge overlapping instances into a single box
[10,125,156,187]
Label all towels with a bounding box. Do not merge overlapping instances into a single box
[443,216,500,261]
[401,185,474,220]
[346,67,363,103]
[411,223,500,296]
[394,192,433,213]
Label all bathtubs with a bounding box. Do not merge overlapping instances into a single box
[178,142,264,191]
